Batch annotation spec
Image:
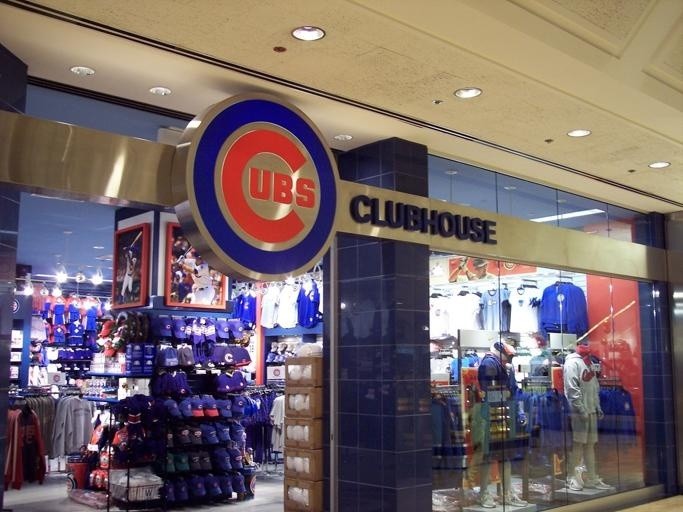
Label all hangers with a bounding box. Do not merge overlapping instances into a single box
[9,386,107,412]
[430,276,568,299]
[243,382,285,398]
[516,378,621,394]
[431,389,457,398]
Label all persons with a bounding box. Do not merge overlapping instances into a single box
[448,256,498,282]
[169,235,225,305]
[115,243,142,303]
[562,343,615,492]
[473,338,531,510]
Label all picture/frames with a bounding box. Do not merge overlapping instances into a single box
[110,222,149,310]
[164,222,226,309]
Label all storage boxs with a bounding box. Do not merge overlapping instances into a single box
[282,355,324,512]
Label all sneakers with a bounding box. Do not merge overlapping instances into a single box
[586,475,610,489]
[498,491,528,507]
[565,475,582,490]
[477,491,496,508]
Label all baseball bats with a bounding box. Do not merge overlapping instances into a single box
[127,231,143,248]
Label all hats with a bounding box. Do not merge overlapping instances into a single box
[167,449,243,471]
[157,370,247,393]
[177,422,244,443]
[162,344,252,368]
[164,472,247,501]
[156,317,243,342]
[165,396,245,418]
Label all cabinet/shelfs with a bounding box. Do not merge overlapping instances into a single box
[81,370,150,402]
[260,321,322,382]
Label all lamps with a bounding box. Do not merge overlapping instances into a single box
[15,273,112,297]
